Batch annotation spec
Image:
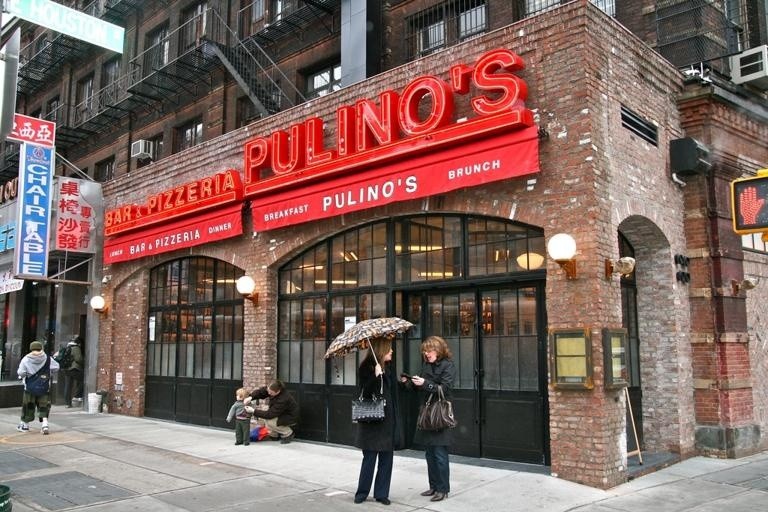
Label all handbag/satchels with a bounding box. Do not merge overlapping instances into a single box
[415,385,457,430]
[25,354,50,396]
[352,386,386,424]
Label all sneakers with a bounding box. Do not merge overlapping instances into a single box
[282,433,295,444]
[235,442,242,445]
[245,442,249,445]
[17,424,29,432]
[71,397,83,402]
[40,425,49,435]
[262,435,279,440]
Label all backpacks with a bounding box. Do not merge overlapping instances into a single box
[62,345,80,368]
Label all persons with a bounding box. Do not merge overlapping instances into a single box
[17,341,60,435]
[226,387,258,445]
[354,337,405,505]
[62,337,84,408]
[243,380,300,444]
[400,336,457,501]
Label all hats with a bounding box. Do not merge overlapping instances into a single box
[30,341,42,350]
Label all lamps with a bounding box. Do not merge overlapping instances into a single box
[548,233,576,281]
[236,276,259,307]
[90,295,108,318]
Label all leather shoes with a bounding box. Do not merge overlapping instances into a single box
[376,498,390,504]
[355,498,366,502]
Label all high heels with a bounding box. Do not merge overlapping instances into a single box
[421,489,435,495]
[431,492,449,502]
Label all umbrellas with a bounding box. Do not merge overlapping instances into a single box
[323,316,417,375]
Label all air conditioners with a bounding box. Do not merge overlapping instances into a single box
[729,45,768,85]
[131,140,153,159]
